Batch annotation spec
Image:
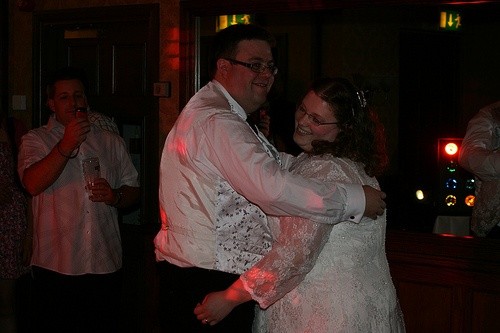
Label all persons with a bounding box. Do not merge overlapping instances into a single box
[142,24,406,333]
[0,71,141,333]
[457,100,500,238]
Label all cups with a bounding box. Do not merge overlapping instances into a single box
[82,158,99,196]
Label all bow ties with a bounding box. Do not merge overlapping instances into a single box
[246,115,259,135]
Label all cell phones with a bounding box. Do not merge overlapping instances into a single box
[75,106,87,120]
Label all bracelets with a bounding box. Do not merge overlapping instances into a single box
[57,139,79,159]
[105,189,122,208]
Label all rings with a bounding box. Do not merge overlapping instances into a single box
[205,318,208,325]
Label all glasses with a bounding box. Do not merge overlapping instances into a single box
[295,104,343,127]
[227,57,280,76]
